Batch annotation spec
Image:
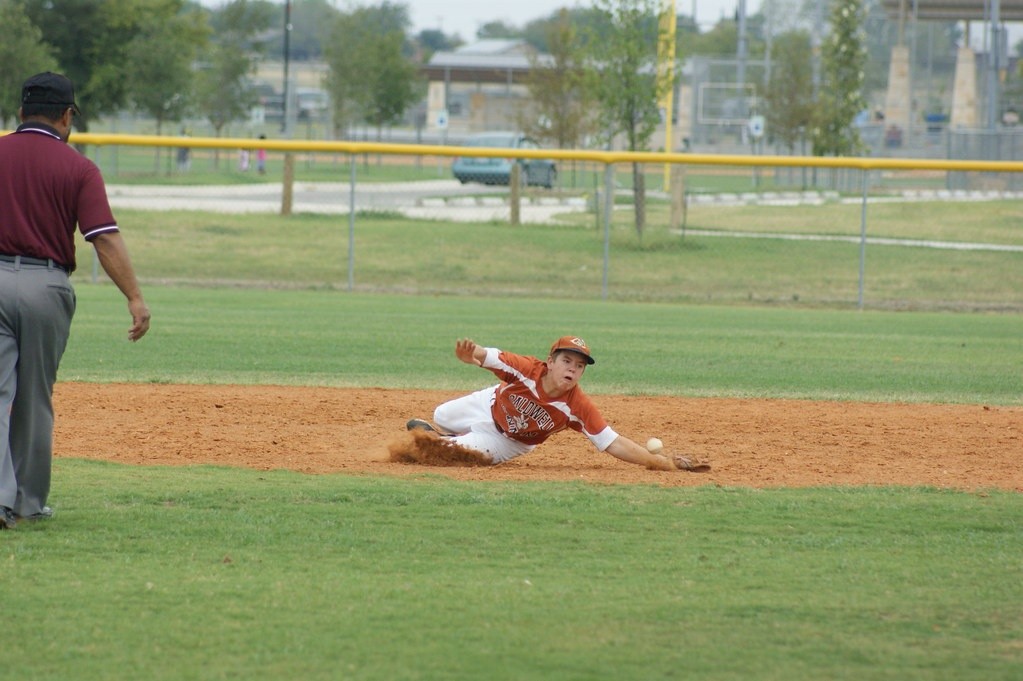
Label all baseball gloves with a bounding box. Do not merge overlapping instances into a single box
[650,456,713,473]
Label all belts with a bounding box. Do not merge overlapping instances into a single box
[0,253,69,272]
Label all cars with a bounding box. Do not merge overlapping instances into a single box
[450,132,557,190]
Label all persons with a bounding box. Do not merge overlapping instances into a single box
[874,105,885,120]
[406,336,712,473]
[239,148,250,172]
[0,70,150,531]
[256,135,267,174]
[885,124,902,148]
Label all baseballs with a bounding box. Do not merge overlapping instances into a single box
[647,438,663,454]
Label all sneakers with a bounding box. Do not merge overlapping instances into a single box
[406,418,456,440]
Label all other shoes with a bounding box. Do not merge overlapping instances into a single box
[26,507,52,519]
[0,504,17,530]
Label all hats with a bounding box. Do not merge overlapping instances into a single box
[21,70,82,116]
[550,335,595,365]
